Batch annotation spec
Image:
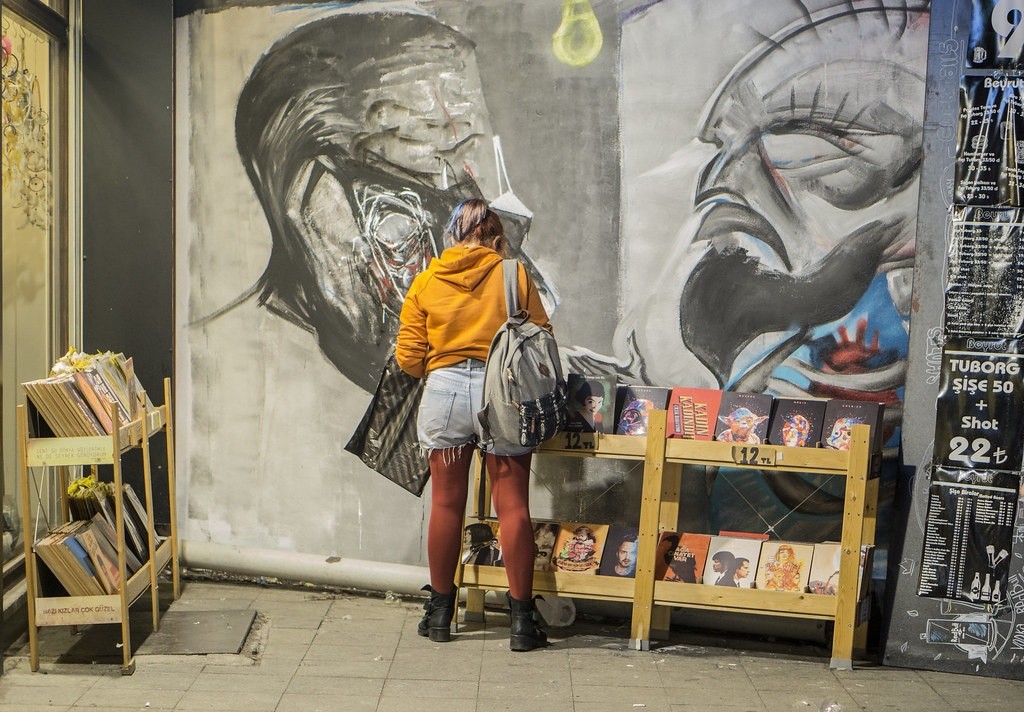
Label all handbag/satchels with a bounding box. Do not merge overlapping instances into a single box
[343,349,431,498]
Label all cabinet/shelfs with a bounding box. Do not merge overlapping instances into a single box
[452,407,882,670]
[19,379,182,676]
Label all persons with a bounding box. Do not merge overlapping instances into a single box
[559,525,597,562]
[395,198,553,652]
[463,524,504,567]
[565,381,604,433]
[712,551,750,588]
[601,534,637,578]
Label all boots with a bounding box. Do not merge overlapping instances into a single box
[415,585,457,642]
[504,591,548,652]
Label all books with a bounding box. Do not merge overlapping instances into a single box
[21,350,154,438]
[32,476,162,596]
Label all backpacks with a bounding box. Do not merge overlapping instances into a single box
[477,259,566,458]
